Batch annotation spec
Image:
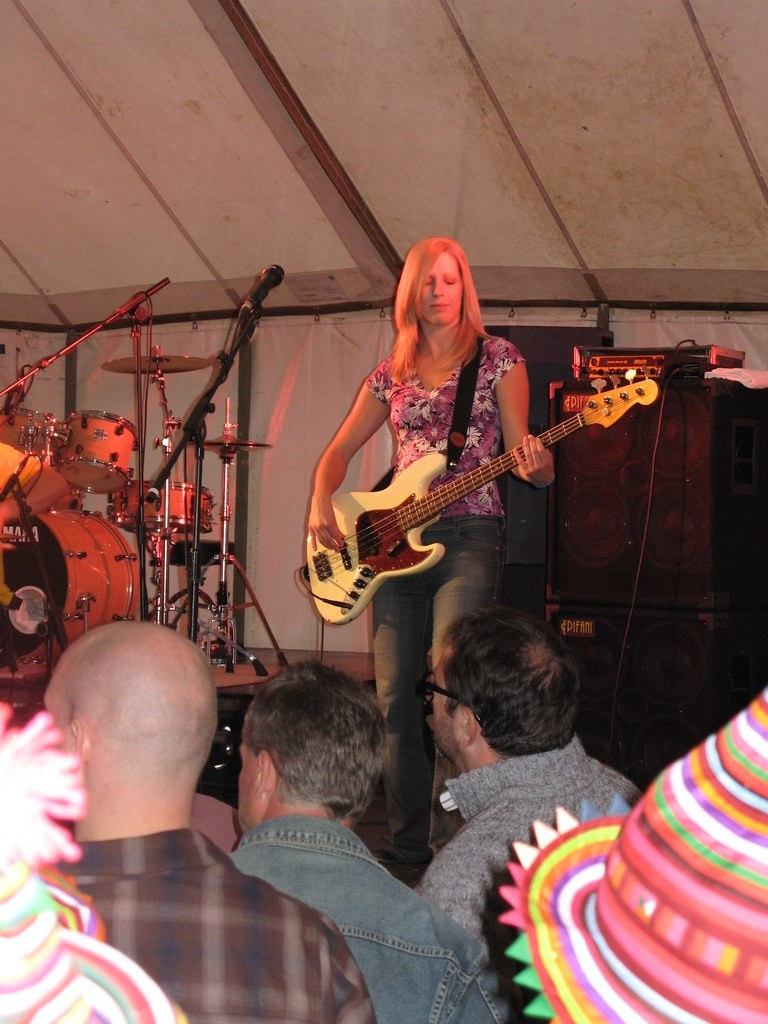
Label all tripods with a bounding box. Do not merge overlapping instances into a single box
[145,305,289,676]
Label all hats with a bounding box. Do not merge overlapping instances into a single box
[500,685,768,1024]
[0,699,193,1024]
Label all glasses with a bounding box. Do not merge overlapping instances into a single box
[413,668,484,724]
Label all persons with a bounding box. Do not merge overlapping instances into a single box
[309,241,557,893]
[1,613,768,1024]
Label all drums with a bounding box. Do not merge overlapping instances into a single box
[111,479,213,534]
[53,409,138,494]
[0,406,72,471]
[0,508,139,662]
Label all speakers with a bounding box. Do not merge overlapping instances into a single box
[545,378,768,610]
[545,602,768,791]
[484,325,614,567]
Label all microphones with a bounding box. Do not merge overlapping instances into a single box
[239,264,284,315]
[10,369,25,405]
[0,456,29,502]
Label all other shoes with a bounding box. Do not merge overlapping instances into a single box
[374,846,434,871]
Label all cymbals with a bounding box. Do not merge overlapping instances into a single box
[100,355,213,375]
[188,435,273,453]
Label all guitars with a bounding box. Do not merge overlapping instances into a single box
[306,368,660,626]
